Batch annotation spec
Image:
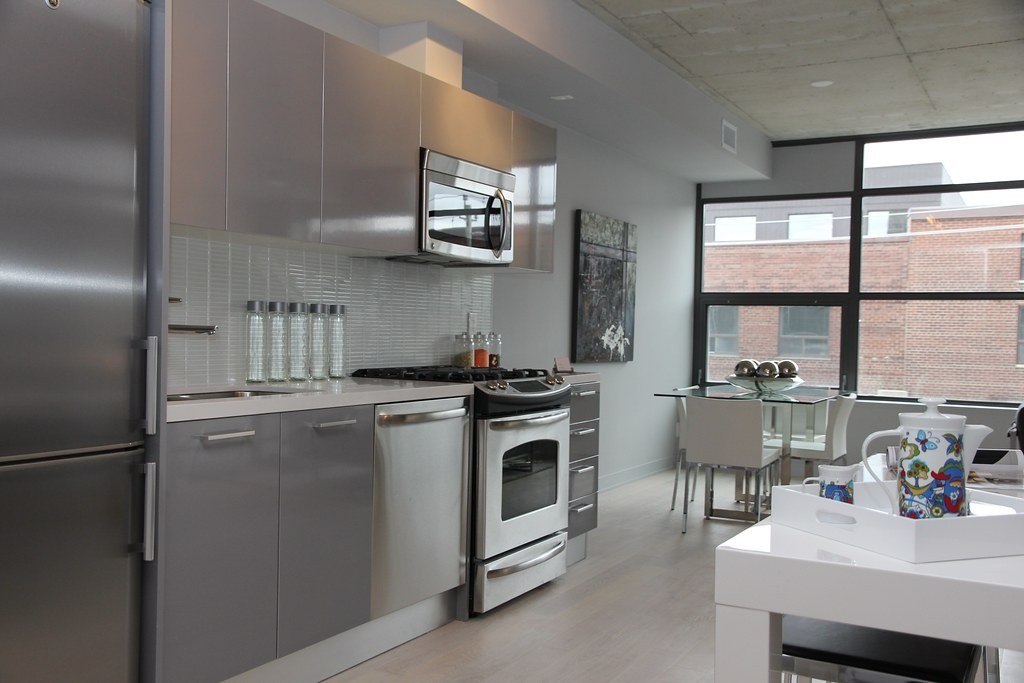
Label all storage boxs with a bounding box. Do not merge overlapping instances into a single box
[770,481,1024,564]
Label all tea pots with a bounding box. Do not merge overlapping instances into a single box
[860,397,993,521]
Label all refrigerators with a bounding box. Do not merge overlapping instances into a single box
[1,1,160,683]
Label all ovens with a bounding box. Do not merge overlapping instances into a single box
[470,408,569,618]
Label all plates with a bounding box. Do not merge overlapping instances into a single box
[725,377,803,392]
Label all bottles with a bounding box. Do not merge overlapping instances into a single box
[247,300,347,382]
[453,331,502,368]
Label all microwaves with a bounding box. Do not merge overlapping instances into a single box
[376,145,516,270]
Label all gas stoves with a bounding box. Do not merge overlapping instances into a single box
[350,365,571,418]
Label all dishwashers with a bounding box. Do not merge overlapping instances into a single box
[369,396,471,626]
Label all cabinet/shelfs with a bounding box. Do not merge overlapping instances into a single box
[166,402,372,683]
[171,0,324,252]
[568,381,601,569]
[511,111,557,276]
[421,70,512,174]
[324,28,424,257]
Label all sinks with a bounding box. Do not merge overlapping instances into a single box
[167,391,292,401]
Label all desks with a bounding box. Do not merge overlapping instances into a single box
[652,385,858,521]
[715,453,1024,683]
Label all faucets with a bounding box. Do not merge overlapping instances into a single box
[168,297,219,336]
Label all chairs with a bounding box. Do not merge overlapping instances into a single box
[671,386,857,535]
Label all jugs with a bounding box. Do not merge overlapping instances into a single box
[801,464,865,505]
